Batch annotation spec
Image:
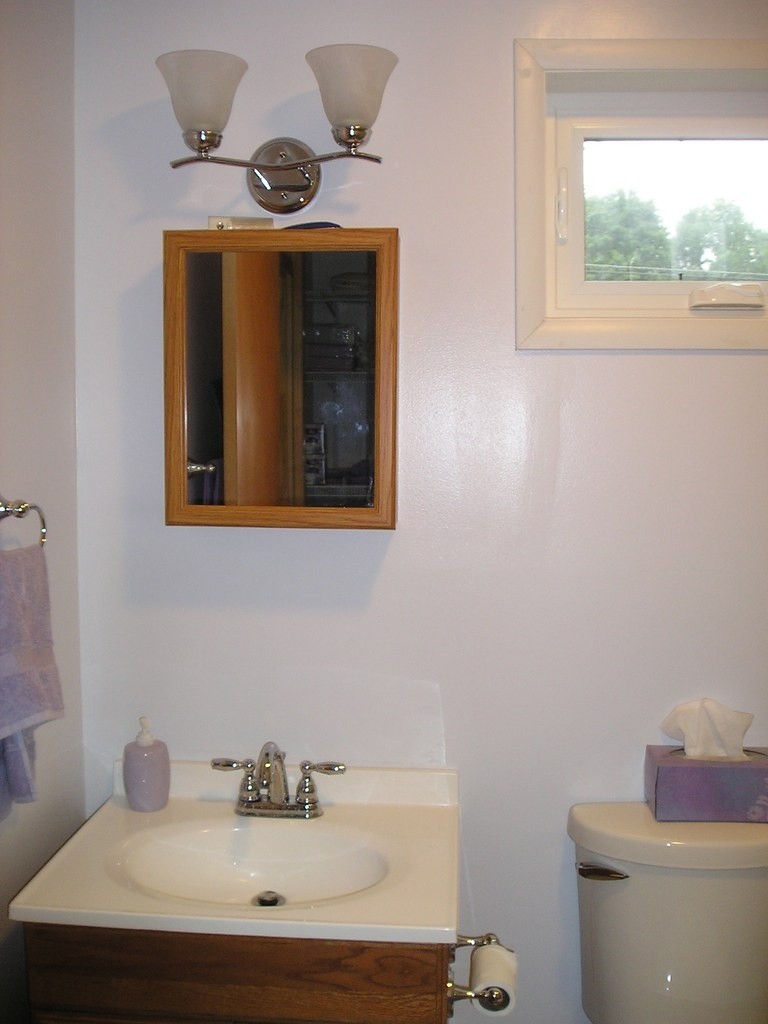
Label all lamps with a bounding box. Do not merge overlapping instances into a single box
[153,42,402,214]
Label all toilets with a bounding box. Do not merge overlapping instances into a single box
[564,798,766,1024]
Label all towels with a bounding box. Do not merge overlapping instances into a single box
[0,543,71,828]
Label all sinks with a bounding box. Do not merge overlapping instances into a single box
[6,790,463,949]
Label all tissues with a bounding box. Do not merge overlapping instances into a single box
[642,700,768,826]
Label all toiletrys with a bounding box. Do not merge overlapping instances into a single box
[121,713,174,814]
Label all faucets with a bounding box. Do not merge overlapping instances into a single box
[255,740,290,813]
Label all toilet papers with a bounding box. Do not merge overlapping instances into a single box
[466,942,523,1019]
